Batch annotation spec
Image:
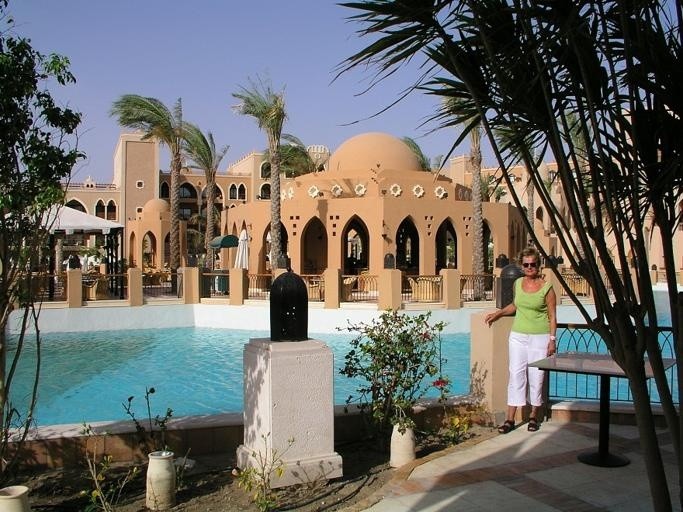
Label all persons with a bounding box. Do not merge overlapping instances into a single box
[485,248,556,434]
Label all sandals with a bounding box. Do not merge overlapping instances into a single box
[498,420,515,433]
[528,418,537,431]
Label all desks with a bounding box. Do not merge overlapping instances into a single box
[526,350,678,468]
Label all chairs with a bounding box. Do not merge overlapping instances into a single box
[0,263,169,301]
[303,271,466,303]
[561,266,590,297]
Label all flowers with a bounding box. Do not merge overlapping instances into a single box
[336,303,454,435]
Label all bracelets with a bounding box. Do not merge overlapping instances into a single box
[550,335,556,341]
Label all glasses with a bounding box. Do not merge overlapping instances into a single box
[523,263,536,267]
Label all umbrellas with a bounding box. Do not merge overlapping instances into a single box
[234,228,248,271]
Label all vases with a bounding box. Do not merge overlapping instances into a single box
[389,417,416,468]
[145,449,177,510]
[0,485,30,511]
